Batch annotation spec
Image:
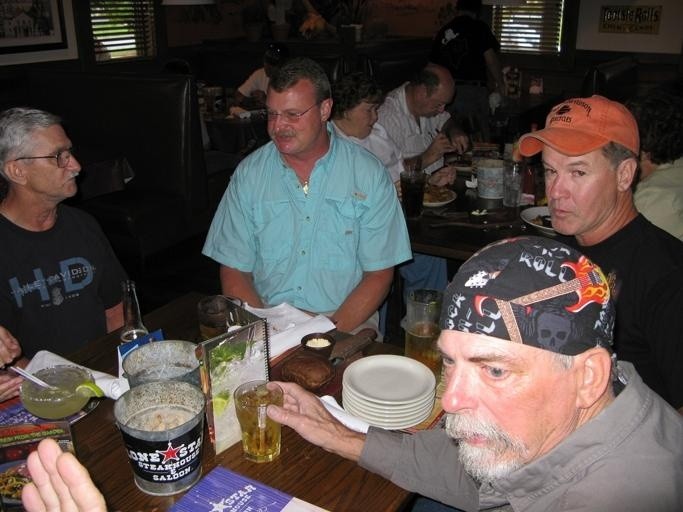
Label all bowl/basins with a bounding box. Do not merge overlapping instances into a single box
[19,365,96,420]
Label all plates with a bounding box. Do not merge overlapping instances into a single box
[422,189,458,208]
[341,353,437,432]
[444,156,472,172]
[519,206,555,236]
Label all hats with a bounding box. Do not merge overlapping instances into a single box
[518,94,641,157]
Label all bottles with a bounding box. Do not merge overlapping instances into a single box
[121,281,148,339]
[523,123,538,200]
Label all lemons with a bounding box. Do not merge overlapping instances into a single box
[75,381,104,399]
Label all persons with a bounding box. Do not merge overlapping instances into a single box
[156,0,682,240]
[0,373,27,405]
[20,437,109,512]
[254,235,683,511]
[201,56,415,334]
[517,92,682,413]
[0,104,129,370]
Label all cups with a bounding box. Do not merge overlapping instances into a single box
[197,294,247,337]
[113,340,207,497]
[502,145,531,208]
[405,291,442,373]
[399,171,426,220]
[233,379,284,464]
[300,333,336,360]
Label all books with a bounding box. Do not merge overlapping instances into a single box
[169,464,332,512]
[199,293,272,456]
[0,420,77,505]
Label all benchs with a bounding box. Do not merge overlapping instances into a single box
[8,66,198,304]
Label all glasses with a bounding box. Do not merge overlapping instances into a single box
[14,149,70,168]
[258,102,317,123]
